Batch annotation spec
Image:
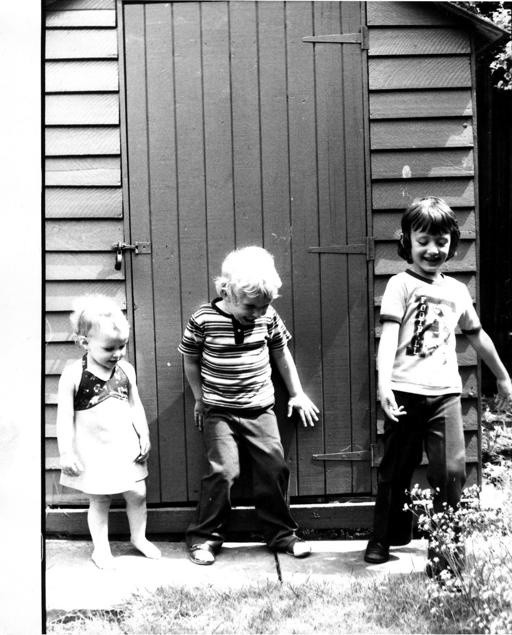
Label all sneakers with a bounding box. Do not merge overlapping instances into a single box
[281,538,311,558]
[188,543,217,566]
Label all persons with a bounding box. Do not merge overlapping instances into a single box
[55,303,162,570]
[365,196,512,579]
[176,246,320,565]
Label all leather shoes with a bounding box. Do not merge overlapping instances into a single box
[364,540,392,564]
[425,561,467,593]
